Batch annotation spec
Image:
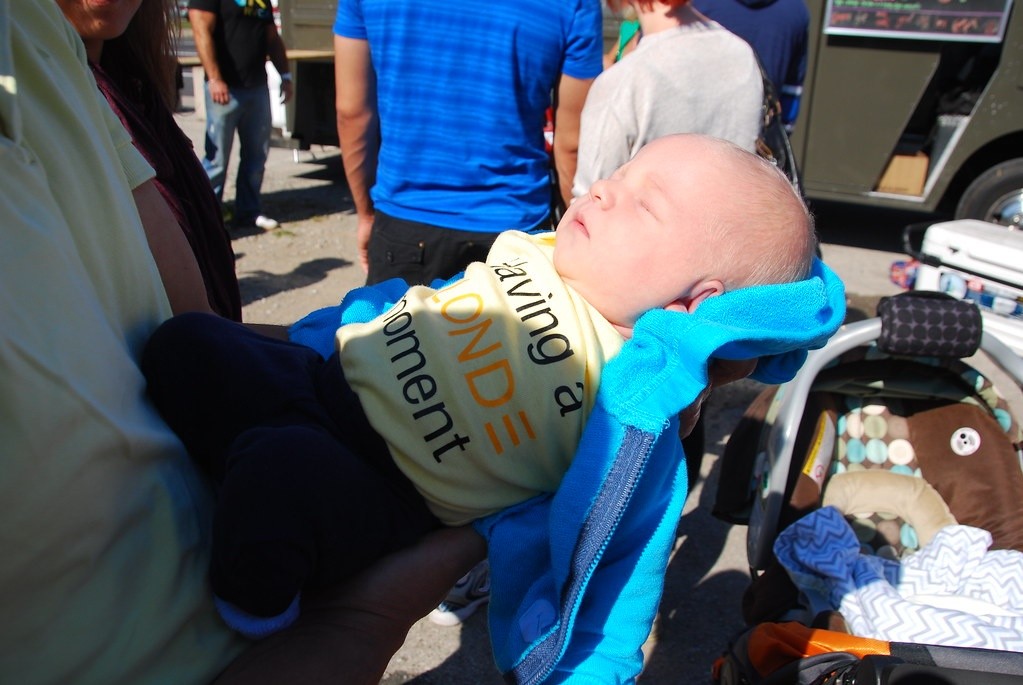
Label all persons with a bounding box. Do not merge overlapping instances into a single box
[186,0,295,229]
[57,0,244,323]
[571,0,766,205]
[691,0,810,171]
[0,0,760,685]
[142,132,815,575]
[332,0,605,288]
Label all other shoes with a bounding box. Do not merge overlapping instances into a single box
[239,214,279,229]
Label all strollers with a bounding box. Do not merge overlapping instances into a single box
[710,291,1023,685]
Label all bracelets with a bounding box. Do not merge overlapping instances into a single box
[281,72,291,80]
